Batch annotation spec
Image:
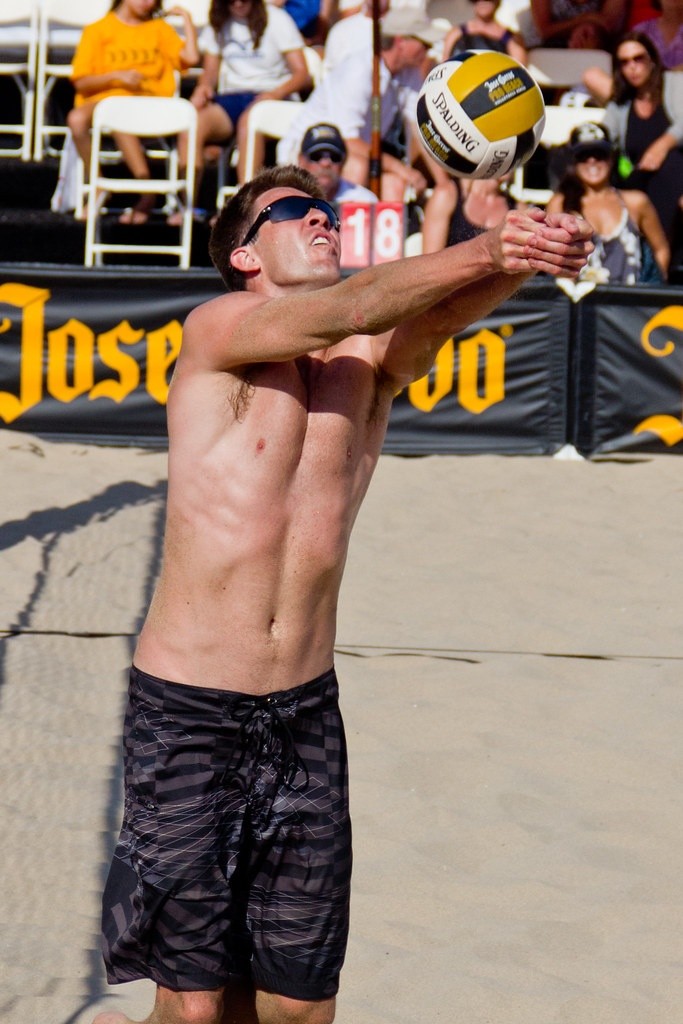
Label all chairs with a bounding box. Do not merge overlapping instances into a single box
[0,1,618,269]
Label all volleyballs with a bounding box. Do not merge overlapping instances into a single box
[412,48,547,181]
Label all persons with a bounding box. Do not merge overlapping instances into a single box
[264,1,530,68]
[598,31,683,270]
[445,168,546,248]
[167,0,310,225]
[545,121,669,285]
[297,123,378,207]
[67,0,201,224]
[277,5,456,254]
[532,0,683,104]
[101,164,596,1024]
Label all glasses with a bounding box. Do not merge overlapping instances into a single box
[379,37,394,51]
[617,54,648,68]
[240,196,343,246]
[310,150,343,162]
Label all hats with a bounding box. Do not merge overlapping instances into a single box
[572,123,614,156]
[380,5,444,43]
[302,124,347,155]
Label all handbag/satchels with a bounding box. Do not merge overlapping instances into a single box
[52,132,84,212]
